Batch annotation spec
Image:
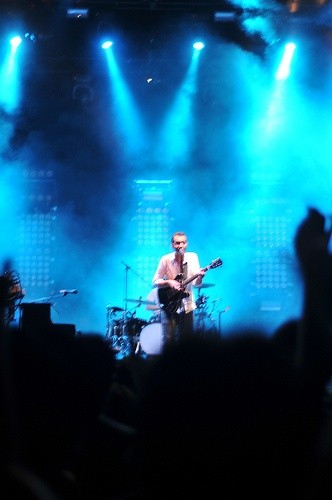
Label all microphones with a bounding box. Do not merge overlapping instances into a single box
[179,248,184,256]
[60,289,78,294]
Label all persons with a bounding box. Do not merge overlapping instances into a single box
[0,208,332,500]
[152,232,204,347]
[146,287,161,315]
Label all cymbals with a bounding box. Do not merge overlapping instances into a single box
[195,284,215,288]
[124,299,156,305]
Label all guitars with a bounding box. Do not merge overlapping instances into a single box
[158,258,223,312]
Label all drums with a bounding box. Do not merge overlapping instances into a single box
[114,318,147,351]
[140,322,163,355]
